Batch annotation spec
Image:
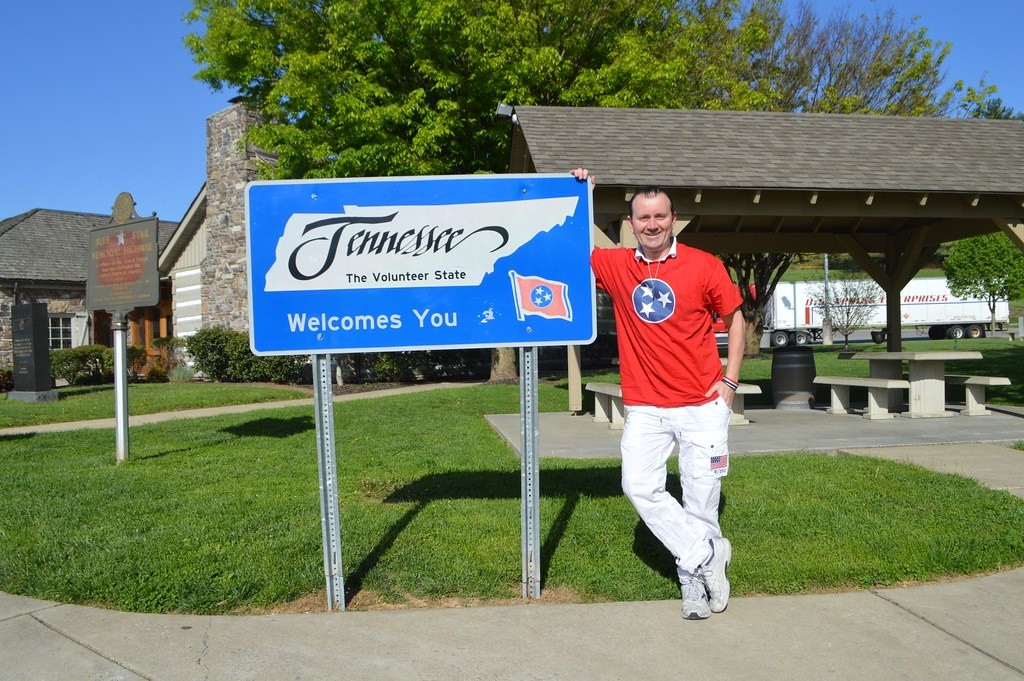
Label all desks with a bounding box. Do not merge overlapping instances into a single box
[838,351,983,418]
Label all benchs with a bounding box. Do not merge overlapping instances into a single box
[945,375,1011,415]
[729,383,762,425]
[585,382,624,429]
[1007,331,1023,341]
[813,376,910,419]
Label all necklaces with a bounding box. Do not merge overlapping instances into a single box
[647,261,660,298]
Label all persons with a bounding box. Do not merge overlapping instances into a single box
[570,168,746,620]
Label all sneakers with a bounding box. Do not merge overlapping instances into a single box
[701,537,731,612]
[677,567,712,619]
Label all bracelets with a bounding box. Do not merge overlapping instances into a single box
[722,376,739,391]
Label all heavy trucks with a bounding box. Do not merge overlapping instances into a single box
[711,277,1011,357]
